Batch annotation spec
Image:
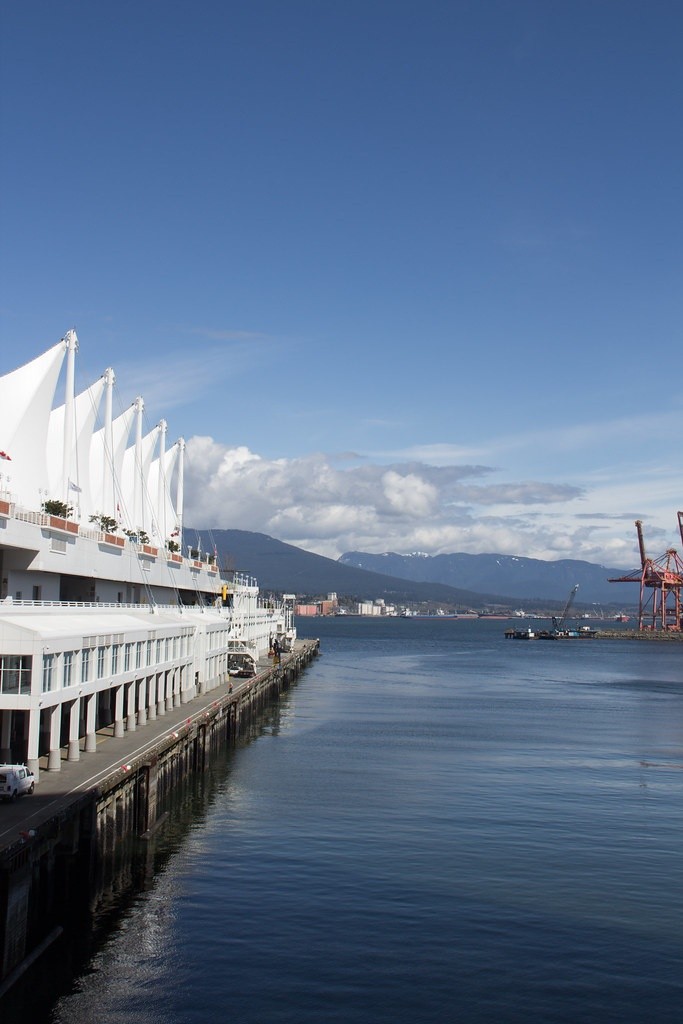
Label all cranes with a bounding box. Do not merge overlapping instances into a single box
[552,583,580,631]
[607,510,683,633]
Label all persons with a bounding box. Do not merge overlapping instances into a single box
[228,682,233,694]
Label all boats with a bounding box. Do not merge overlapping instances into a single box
[616,611,629,622]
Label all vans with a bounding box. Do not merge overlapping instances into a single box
[0,763,35,804]
